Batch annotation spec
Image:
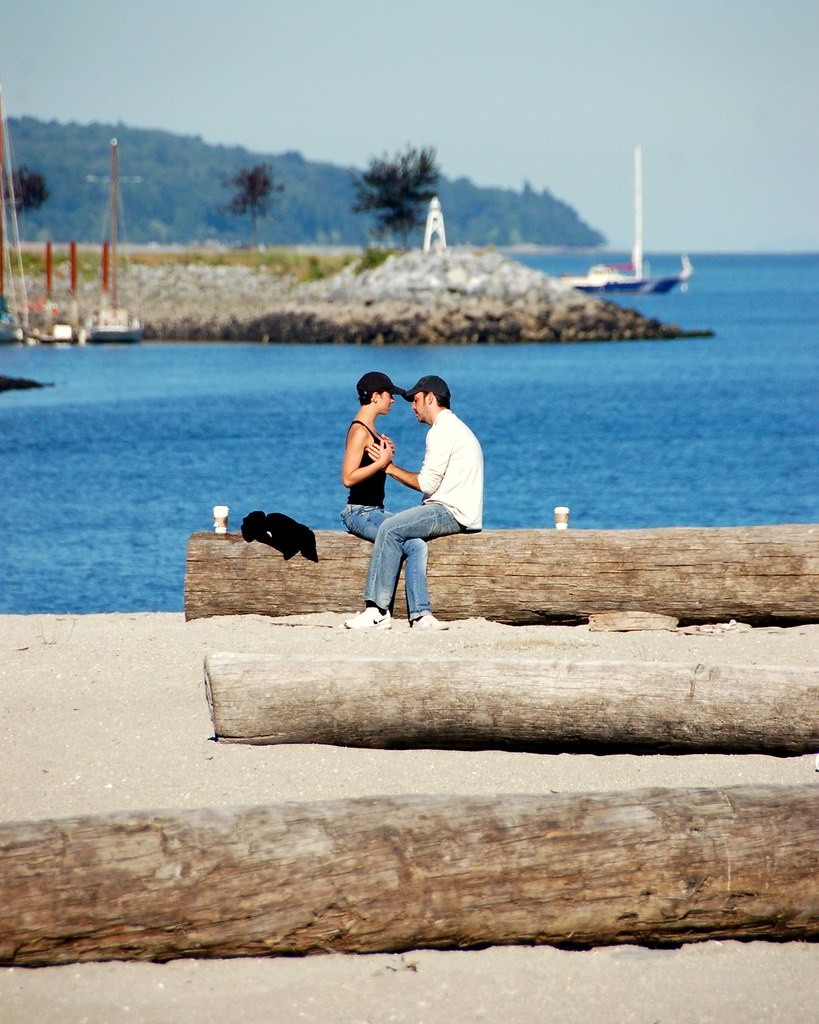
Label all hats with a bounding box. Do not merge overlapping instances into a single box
[401,375,451,402]
[357,372,405,395]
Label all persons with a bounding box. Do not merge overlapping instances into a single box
[345,375,484,629]
[342,371,449,632]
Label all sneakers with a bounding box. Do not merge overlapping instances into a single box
[412,616,449,630]
[345,606,393,629]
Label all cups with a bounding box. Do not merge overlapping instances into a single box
[213,506,230,533]
[554,506,570,530]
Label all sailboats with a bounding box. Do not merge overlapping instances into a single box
[556,139,694,295]
[0,92,146,346]
